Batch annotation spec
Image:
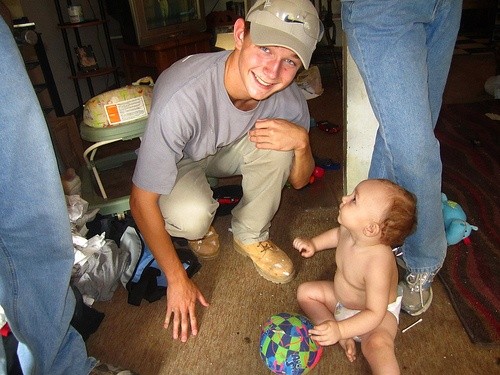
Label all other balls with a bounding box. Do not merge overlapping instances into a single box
[259,313,324,375]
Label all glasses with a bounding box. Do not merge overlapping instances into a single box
[246,0,324,41]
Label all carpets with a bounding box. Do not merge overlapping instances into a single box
[434,99,500,346]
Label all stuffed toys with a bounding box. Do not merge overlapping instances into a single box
[439,191,478,246]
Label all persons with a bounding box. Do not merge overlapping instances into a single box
[129,0,320,341]
[341,0,462,315]
[292,178,417,375]
[0,18,133,375]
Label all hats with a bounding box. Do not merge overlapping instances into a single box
[245,0,325,70]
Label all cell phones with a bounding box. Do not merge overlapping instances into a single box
[317,121,340,134]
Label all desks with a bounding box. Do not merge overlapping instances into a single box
[117,33,213,85]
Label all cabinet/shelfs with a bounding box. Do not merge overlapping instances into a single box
[54,0,121,109]
[18,32,64,119]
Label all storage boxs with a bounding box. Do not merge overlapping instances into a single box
[48,114,87,175]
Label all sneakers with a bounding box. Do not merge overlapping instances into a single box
[398,264,443,316]
[187,226,219,261]
[232,234,295,285]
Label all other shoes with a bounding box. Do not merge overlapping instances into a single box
[90,362,135,375]
